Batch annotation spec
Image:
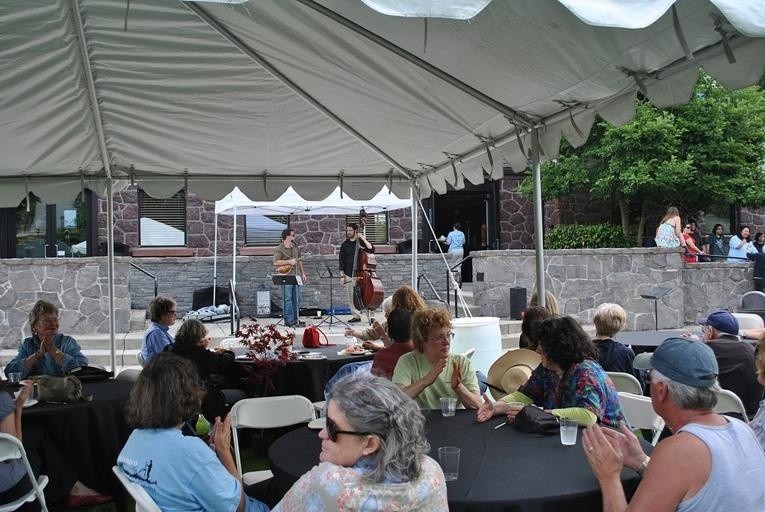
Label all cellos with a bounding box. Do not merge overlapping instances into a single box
[353,210,384,310]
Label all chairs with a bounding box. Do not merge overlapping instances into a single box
[739,288,765,315]
[232,395,316,507]
[219,338,252,347]
[710,389,752,424]
[604,371,644,398]
[609,392,666,447]
[116,368,146,381]
[1,431,50,512]
[632,351,661,385]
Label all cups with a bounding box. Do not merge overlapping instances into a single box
[317,310,322,317]
[21,386,35,402]
[558,416,578,446]
[438,395,459,417]
[435,444,462,483]
[6,371,22,383]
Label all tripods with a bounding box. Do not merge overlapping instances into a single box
[316,277,349,330]
[275,283,297,330]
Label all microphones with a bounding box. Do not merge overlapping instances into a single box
[291,240,297,247]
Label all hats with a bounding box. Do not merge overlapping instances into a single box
[632,338,719,388]
[487,349,543,402]
[698,308,739,334]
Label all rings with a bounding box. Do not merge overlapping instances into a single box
[588,447,593,452]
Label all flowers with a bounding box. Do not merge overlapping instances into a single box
[235,319,294,367]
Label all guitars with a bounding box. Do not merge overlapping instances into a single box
[278,256,304,275]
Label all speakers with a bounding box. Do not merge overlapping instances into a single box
[510,288,527,320]
[192,285,230,311]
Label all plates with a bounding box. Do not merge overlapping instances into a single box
[349,349,373,355]
[300,354,327,360]
[235,355,261,363]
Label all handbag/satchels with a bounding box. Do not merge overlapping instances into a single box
[303,328,319,348]
[36,374,82,402]
[514,405,560,435]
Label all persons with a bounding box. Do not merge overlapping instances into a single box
[653,206,686,248]
[0,379,114,512]
[681,219,765,262]
[476,292,647,435]
[116,352,271,512]
[345,284,482,410]
[139,296,177,361]
[272,229,307,327]
[443,222,466,280]
[581,338,763,511]
[267,372,450,512]
[678,308,764,449]
[3,301,89,380]
[338,223,376,323]
[161,318,235,423]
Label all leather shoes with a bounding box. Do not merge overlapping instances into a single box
[286,321,305,327]
[348,318,360,322]
[67,495,112,508]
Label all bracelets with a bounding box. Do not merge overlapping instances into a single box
[637,455,651,475]
[34,350,42,360]
[53,350,61,358]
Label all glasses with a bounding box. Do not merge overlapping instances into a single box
[326,409,387,442]
[426,333,454,341]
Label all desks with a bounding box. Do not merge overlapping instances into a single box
[609,329,763,365]
[263,408,663,512]
[217,345,387,401]
[0,375,157,502]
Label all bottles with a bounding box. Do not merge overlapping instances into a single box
[360,311,370,331]
[345,327,355,351]
